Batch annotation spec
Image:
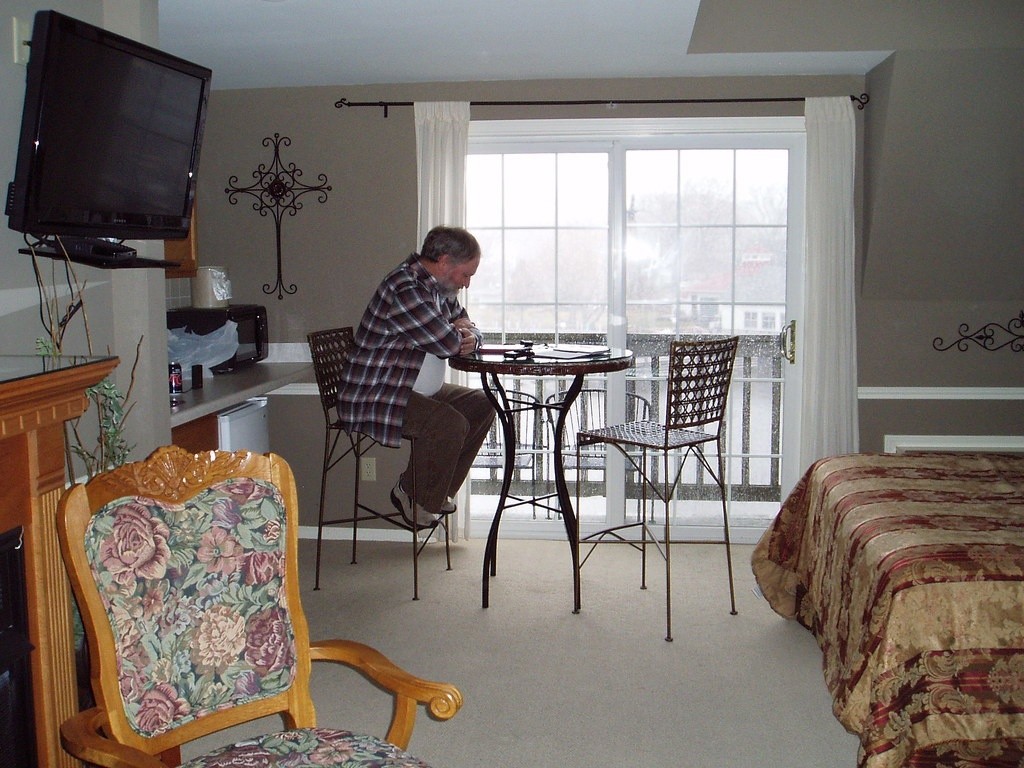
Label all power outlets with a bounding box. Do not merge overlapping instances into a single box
[361,457,377,481]
[14,18,32,66]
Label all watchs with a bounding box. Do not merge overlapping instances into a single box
[474,333,479,354]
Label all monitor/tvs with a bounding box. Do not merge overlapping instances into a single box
[4,9,212,264]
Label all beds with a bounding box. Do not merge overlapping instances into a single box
[751,449,1024,768]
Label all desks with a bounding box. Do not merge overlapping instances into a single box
[170,363,316,457]
[449,345,633,614]
[0,354,122,768]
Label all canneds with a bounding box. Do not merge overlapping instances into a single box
[168,361,182,395]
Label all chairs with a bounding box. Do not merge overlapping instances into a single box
[572,335,739,642]
[55,445,464,768]
[308,326,453,601]
[472,388,543,520]
[545,388,654,521]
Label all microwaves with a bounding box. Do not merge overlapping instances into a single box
[167,303,268,373]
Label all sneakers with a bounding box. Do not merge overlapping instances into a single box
[390,481,438,529]
[433,497,457,515]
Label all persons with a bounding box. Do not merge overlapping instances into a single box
[335,225,495,526]
